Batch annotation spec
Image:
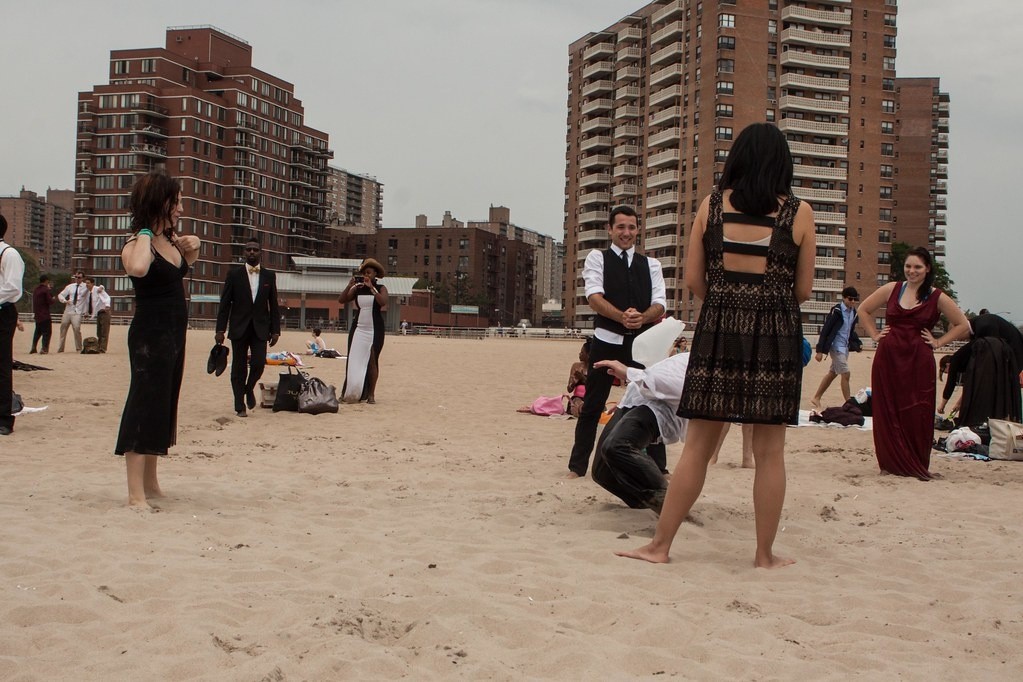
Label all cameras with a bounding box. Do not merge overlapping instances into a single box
[354,277,365,283]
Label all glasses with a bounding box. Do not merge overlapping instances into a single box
[246,247,259,252]
[75,276,81,279]
[847,297,859,301]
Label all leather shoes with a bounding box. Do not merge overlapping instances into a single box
[207,343,229,376]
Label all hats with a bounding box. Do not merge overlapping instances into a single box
[939,355,950,381]
[355,257,385,278]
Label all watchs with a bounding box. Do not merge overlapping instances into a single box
[137,228,154,238]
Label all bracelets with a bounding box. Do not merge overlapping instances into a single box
[569,383,573,390]
[371,287,378,295]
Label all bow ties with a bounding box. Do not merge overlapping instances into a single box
[248,266,260,275]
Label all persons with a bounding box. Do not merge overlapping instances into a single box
[496,320,582,341]
[566,122,816,569]
[811,287,863,409]
[937,309,1023,430]
[305,328,326,356]
[114,172,200,508]
[670,337,689,356]
[214,238,281,417]
[401,319,408,336]
[567,342,608,417]
[29,272,111,354]
[0,214,24,436]
[338,258,389,403]
[857,246,969,481]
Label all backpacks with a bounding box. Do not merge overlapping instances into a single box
[81,337,99,353]
[808,402,865,427]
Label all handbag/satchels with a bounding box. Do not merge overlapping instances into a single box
[531,393,574,415]
[259,365,338,415]
[987,417,1023,460]
[946,427,981,451]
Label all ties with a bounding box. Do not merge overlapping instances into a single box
[73,285,79,305]
[620,250,629,268]
[88,292,92,315]
